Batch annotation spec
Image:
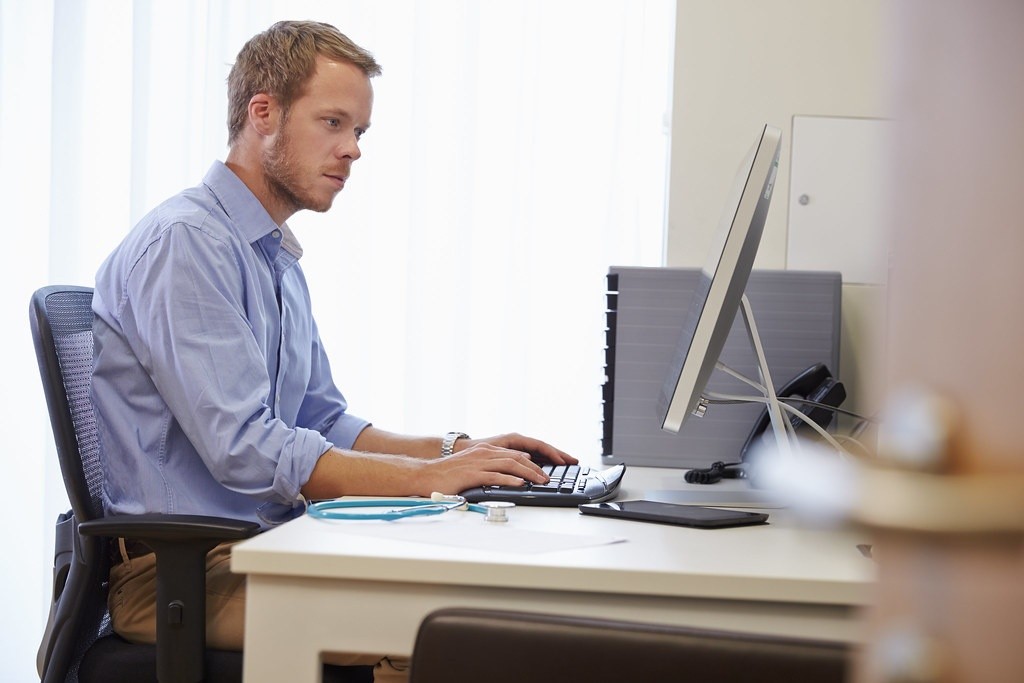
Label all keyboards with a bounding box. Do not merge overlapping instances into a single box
[458,462,626,505]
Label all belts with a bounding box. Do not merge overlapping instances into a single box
[110,539,152,555]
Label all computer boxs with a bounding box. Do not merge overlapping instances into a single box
[600,266,842,469]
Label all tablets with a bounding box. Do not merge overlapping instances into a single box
[578,499,768,528]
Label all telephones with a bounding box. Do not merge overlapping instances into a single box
[739,362,849,471]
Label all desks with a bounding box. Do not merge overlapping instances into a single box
[231,462,874,683]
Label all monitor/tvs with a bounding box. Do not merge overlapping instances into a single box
[660,124,782,435]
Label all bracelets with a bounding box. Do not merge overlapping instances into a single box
[439,431,471,459]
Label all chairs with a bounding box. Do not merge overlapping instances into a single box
[405,608,855,683]
[29,282,376,682]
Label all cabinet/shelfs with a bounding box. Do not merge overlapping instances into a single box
[599,266,844,470]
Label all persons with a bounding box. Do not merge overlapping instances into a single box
[89,18,579,683]
